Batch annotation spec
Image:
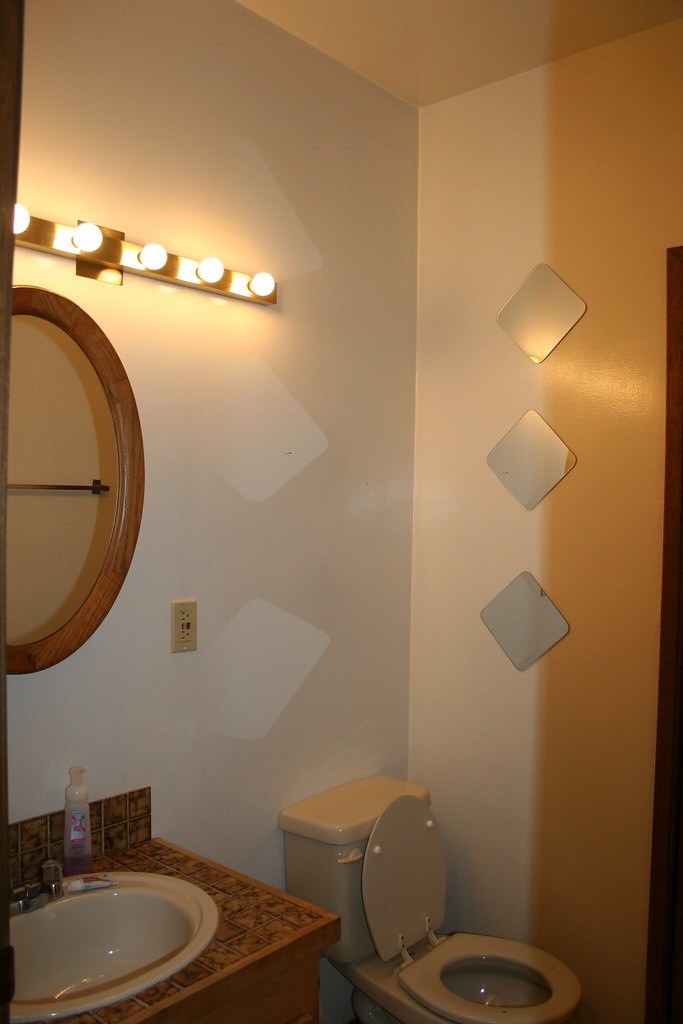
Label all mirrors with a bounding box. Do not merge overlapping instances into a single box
[479,571,568,671]
[3,283,147,677]
[495,263,586,365]
[485,410,577,511]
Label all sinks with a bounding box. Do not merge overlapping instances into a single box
[7,870,220,1017]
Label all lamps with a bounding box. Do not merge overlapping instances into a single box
[12,202,279,306]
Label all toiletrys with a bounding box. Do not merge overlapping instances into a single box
[67,872,121,892]
[59,763,94,862]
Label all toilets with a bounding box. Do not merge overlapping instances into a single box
[276,771,587,1024]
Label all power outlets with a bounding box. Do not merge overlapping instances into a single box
[172,598,197,653]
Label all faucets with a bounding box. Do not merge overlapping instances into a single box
[23,879,63,902]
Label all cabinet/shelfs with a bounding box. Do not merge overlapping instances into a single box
[196,948,320,1024]
